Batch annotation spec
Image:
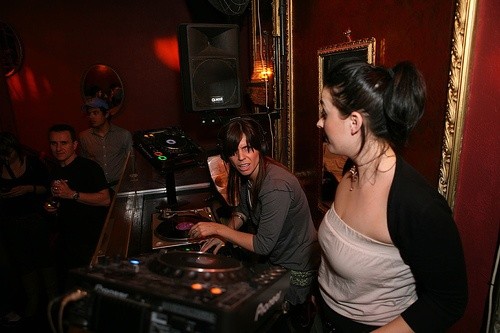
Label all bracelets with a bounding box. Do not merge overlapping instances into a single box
[33,185,37,193]
[73,191,80,201]
[233,213,247,225]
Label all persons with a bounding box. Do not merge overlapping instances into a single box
[0,129,50,199]
[316,59,473,333]
[78,98,135,196]
[1,124,112,329]
[192,118,316,319]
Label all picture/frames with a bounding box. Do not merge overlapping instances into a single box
[318,37,376,212]
[271,0,294,171]
[439,0,500,333]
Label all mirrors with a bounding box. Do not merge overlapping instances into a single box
[81,64,124,118]
[0,22,23,78]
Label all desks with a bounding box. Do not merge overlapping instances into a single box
[90,155,232,271]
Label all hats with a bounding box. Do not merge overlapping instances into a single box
[80,98,108,109]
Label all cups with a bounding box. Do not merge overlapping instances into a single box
[42,186,59,212]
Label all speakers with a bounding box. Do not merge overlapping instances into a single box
[177,23,241,112]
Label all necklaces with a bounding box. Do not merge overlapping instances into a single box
[347,144,391,191]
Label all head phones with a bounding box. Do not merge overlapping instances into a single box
[216,117,269,154]
